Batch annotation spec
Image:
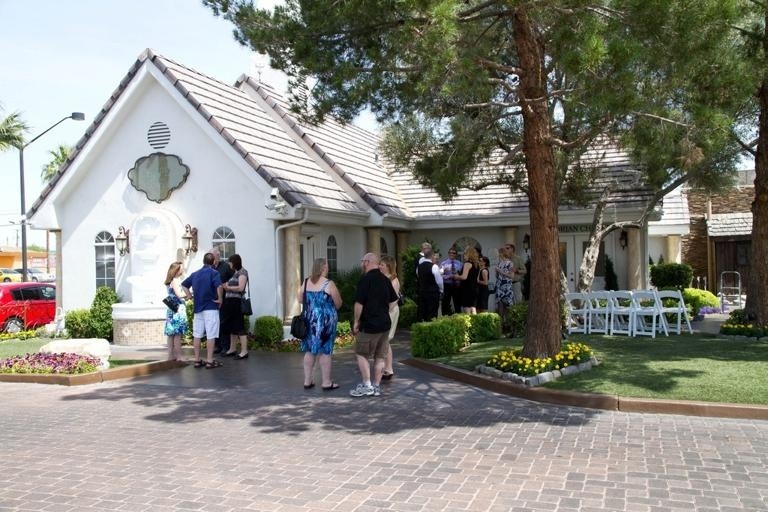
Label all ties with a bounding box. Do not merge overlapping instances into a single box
[451,261,455,273]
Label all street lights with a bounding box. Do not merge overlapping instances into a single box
[18,112,85,282]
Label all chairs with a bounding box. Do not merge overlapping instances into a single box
[714,267,745,315]
[563,283,693,340]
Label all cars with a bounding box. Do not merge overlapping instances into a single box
[0,268,32,283]
[12,267,56,283]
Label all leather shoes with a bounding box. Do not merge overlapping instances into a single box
[221,349,248,360]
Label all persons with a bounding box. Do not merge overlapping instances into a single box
[180,252,224,369]
[349,252,400,397]
[298,258,344,391]
[163,262,192,362]
[378,255,400,381]
[220,254,249,360]
[413,242,532,322]
[208,249,235,356]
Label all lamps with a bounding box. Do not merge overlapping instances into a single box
[114,224,130,256]
[521,232,530,254]
[182,223,197,257]
[617,230,626,250]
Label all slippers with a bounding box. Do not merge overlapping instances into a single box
[323,382,339,390]
[304,380,315,389]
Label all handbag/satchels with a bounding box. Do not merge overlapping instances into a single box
[241,295,252,316]
[290,314,309,340]
[398,290,406,307]
[163,296,178,312]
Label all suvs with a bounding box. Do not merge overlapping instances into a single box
[0,282,56,334]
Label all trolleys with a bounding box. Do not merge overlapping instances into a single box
[720,271,742,314]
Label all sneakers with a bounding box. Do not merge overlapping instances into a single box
[372,383,381,396]
[383,368,393,379]
[349,384,374,397]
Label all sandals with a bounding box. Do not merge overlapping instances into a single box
[194,360,206,368]
[205,361,223,369]
